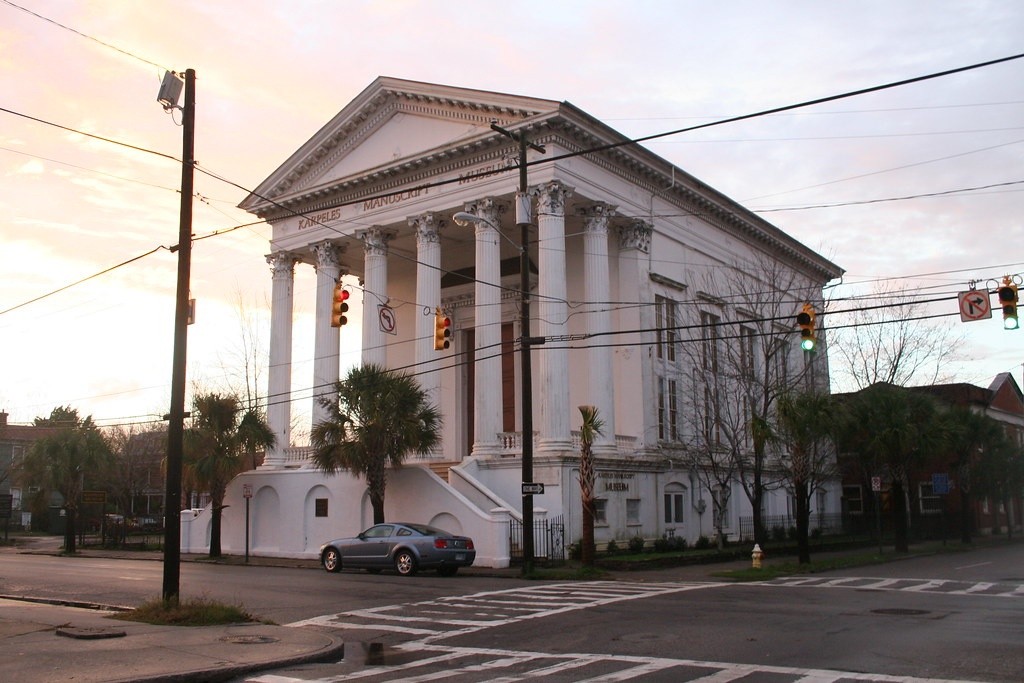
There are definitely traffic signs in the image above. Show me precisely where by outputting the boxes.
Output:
[522,481,544,495]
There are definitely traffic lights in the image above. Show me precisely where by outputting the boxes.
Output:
[330,287,349,328]
[797,305,816,351]
[434,316,450,351]
[998,285,1019,330]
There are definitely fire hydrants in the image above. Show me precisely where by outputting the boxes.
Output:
[751,543,764,568]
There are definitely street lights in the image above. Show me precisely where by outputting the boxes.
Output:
[453,211,534,573]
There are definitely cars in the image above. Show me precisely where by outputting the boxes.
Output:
[90,513,138,532]
[319,523,476,576]
